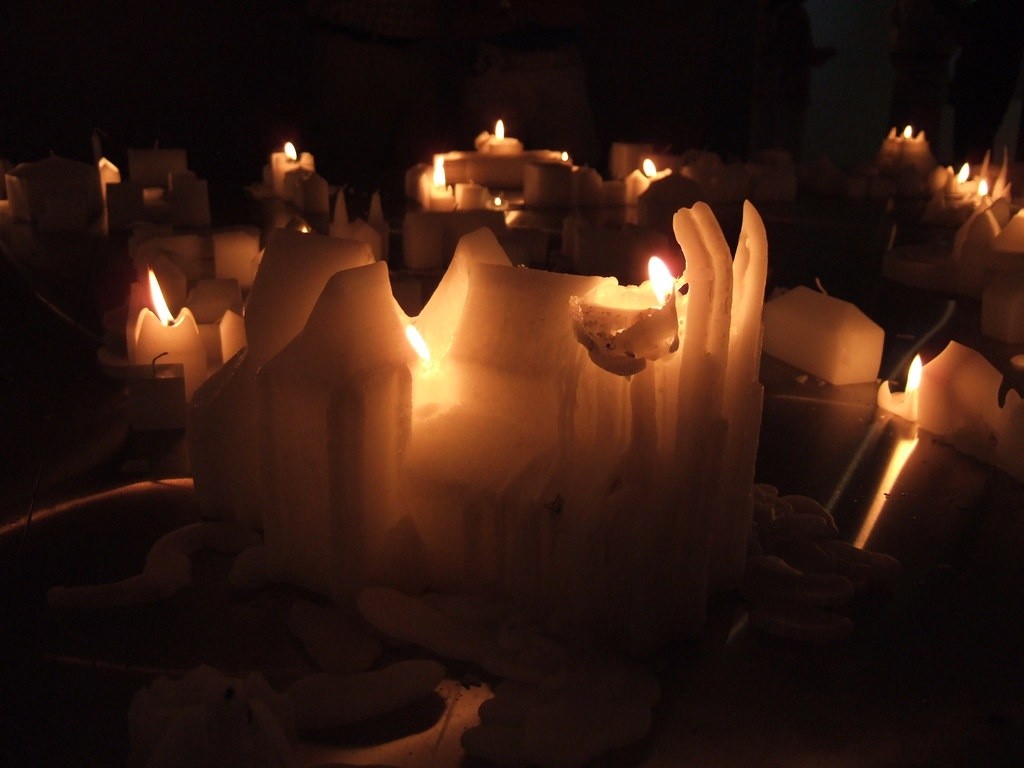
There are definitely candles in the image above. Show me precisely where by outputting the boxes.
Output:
[0,116,1024,661]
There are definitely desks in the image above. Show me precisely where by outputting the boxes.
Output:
[0,472,1024,768]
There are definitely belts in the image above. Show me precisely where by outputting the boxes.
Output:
[316,22,426,47]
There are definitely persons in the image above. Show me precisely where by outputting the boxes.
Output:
[302,0,603,191]
[883,0,1024,166]
[747,0,837,163]
